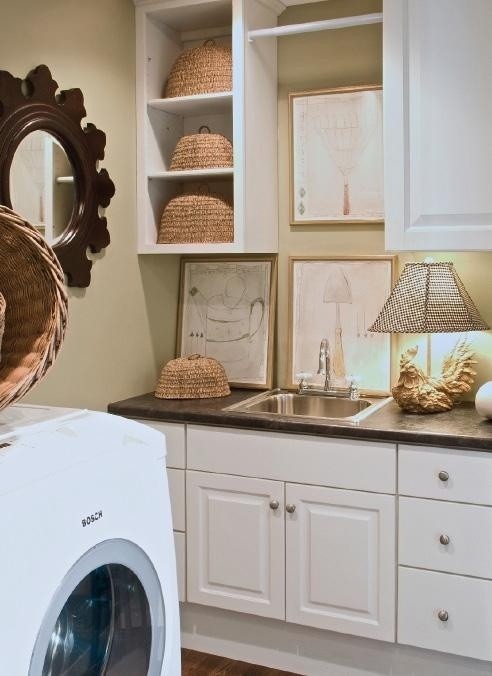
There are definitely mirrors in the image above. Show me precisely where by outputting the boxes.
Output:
[1,65,116,288]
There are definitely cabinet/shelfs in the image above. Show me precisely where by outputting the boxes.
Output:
[384,3,492,254]
[186,422,397,675]
[135,2,279,255]
[396,443,491,663]
[108,395,186,646]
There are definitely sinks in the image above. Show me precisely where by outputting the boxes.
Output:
[222,389,393,422]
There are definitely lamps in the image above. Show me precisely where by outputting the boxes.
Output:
[368,263,491,378]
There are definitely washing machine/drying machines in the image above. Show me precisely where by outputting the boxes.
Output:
[1,401,184,676]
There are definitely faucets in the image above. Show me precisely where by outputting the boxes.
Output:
[297,337,361,399]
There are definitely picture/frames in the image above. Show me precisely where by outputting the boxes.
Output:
[176,257,276,389]
[286,255,400,398]
[288,84,383,225]
